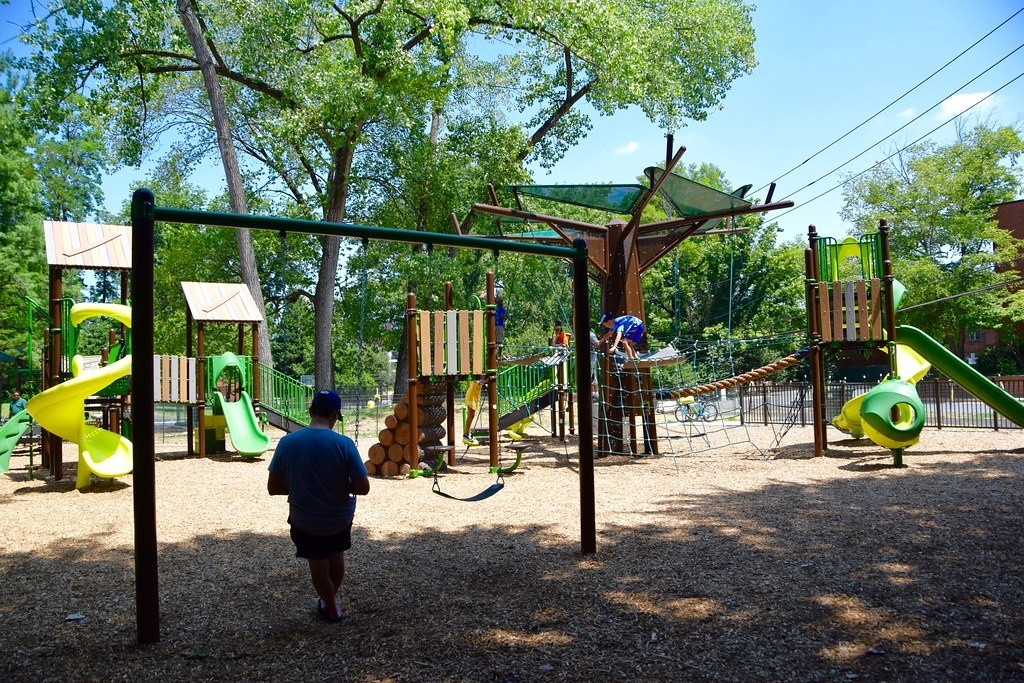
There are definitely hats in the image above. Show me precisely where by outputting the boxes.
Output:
[313,390,342,421]
[598,314,613,325]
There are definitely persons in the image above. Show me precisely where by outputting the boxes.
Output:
[267,390,370,620]
[462,381,482,445]
[598,315,644,359]
[551,326,568,389]
[495,297,509,356]
[589,325,599,402]
[9,391,27,419]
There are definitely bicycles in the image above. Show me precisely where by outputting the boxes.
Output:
[674,395,719,423]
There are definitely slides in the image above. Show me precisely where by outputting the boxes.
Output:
[212,390,271,455]
[833,328,931,449]
[897,323,1024,428]
[26,302,132,479]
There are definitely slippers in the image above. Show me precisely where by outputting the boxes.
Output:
[315,597,343,622]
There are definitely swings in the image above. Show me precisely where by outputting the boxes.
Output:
[280,244,367,553]
[428,254,505,503]
